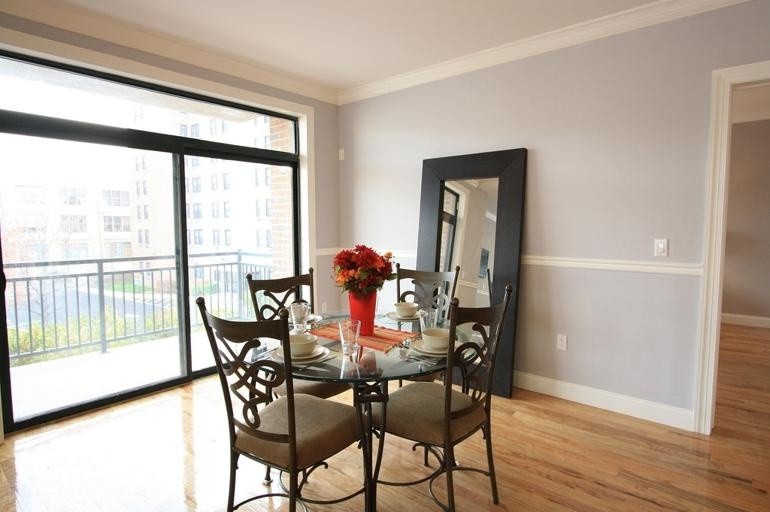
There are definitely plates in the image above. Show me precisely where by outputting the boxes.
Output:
[387,309,429,323]
[272,314,322,326]
[410,340,467,359]
[270,345,330,365]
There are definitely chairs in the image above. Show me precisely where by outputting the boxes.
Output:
[194,296,366,512]
[364,285,512,510]
[247,268,355,404]
[395,263,460,388]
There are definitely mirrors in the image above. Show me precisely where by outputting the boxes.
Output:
[410,148,527,399]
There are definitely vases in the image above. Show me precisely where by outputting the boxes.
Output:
[348,290,378,337]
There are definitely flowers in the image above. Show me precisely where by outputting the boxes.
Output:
[330,245,396,292]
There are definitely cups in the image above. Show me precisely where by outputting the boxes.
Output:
[289,301,312,329]
[336,318,362,355]
[340,352,360,380]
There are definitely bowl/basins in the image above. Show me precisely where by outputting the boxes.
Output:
[393,302,418,317]
[284,333,318,355]
[420,328,455,347]
